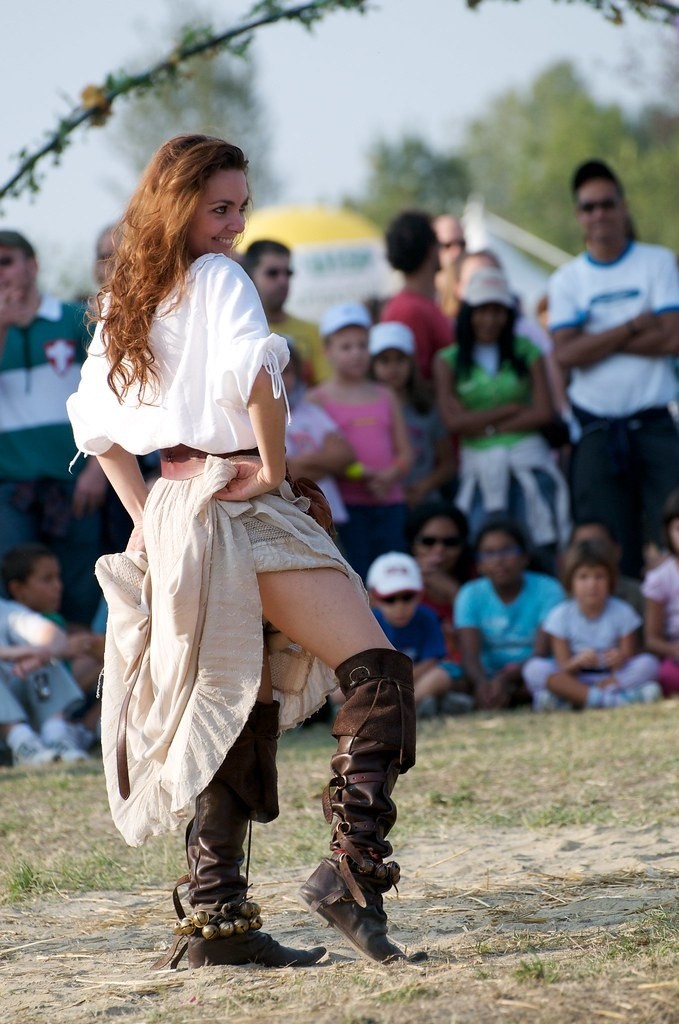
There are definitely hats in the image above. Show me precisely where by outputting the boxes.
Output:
[364,551,422,598]
[315,299,371,339]
[365,321,416,356]
[453,268,516,307]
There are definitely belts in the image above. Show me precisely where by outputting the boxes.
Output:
[629,406,669,424]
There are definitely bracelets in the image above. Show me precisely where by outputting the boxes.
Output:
[625,320,639,335]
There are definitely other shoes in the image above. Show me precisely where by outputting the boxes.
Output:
[13,740,59,767]
[47,735,87,763]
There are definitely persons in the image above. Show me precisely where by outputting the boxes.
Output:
[63,134,431,970]
[0,158,679,769]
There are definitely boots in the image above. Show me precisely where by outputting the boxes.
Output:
[297,647,430,969]
[149,700,327,975]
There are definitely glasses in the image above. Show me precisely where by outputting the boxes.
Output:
[576,197,616,214]
[382,590,418,604]
[416,531,462,548]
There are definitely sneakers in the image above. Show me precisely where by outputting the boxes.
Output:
[535,691,574,712]
[622,685,659,704]
